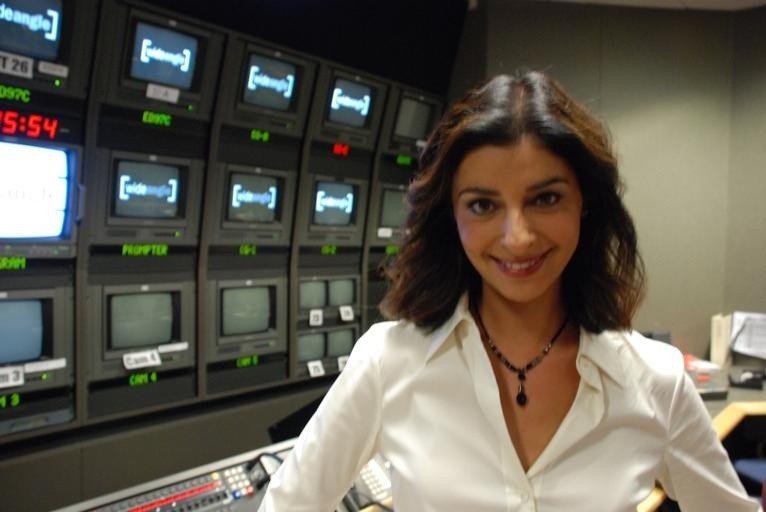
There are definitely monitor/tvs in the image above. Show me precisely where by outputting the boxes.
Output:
[128,20,200,91]
[326,76,373,128]
[100,282,191,361]
[0,141,68,239]
[0,0,65,61]
[114,160,182,218]
[0,285,68,372]
[394,97,432,139]
[242,52,298,111]
[296,271,360,366]
[217,277,280,344]
[227,173,279,223]
[381,189,408,228]
[313,181,355,226]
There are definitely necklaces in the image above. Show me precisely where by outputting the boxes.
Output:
[478,310,566,406]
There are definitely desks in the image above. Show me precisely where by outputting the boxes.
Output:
[640,362,766,512]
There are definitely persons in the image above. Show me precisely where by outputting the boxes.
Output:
[257,69,761,512]
